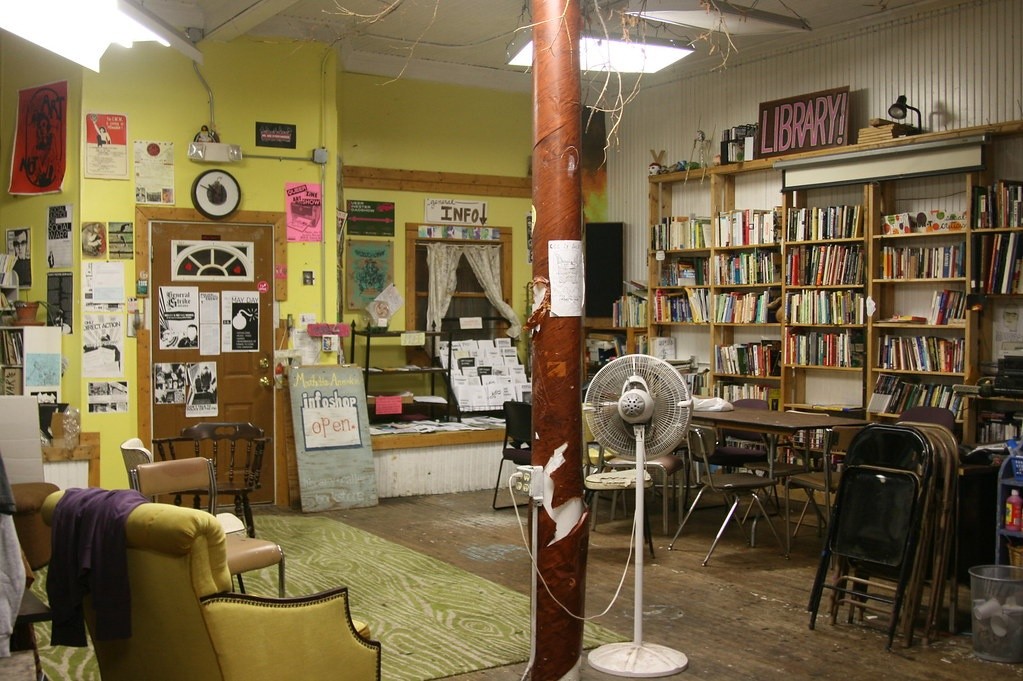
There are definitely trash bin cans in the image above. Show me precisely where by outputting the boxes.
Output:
[968,565,1023,662]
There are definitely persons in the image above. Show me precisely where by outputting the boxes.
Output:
[12,229,31,285]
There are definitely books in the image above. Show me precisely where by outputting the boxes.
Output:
[583,333,781,459]
[928,289,967,325]
[971,231,1023,295]
[879,241,966,279]
[876,335,965,373]
[972,178,1023,229]
[719,122,759,165]
[785,244,864,285]
[866,373,964,420]
[439,338,531,411]
[714,247,781,285]
[714,205,782,247]
[786,403,862,471]
[885,316,926,323]
[786,289,866,325]
[784,326,864,368]
[975,410,1023,445]
[612,213,712,327]
[786,204,863,242]
[713,287,781,324]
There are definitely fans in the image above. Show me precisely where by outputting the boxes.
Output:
[586,356,693,677]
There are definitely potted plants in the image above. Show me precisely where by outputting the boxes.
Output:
[7,298,60,330]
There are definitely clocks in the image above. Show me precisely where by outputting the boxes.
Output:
[190,169,241,219]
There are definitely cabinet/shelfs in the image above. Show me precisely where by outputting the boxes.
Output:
[994,444,1023,587]
[647,120,1023,525]
[0,285,61,403]
[349,321,453,424]
[585,326,647,381]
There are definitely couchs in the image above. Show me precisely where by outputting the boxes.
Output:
[41,490,380,681]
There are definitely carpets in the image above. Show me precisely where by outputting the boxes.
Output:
[29,516,632,681]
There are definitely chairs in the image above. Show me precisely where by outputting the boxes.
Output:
[492,399,960,650]
[130,457,285,598]
[120,437,158,504]
[151,422,272,538]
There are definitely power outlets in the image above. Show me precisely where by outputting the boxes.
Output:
[514,465,543,499]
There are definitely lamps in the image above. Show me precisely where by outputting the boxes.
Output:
[888,95,927,136]
[188,142,242,162]
[506,0,811,74]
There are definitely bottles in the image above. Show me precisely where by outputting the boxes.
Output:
[1005,489,1021,531]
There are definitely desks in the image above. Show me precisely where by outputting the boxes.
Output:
[590,395,873,555]
